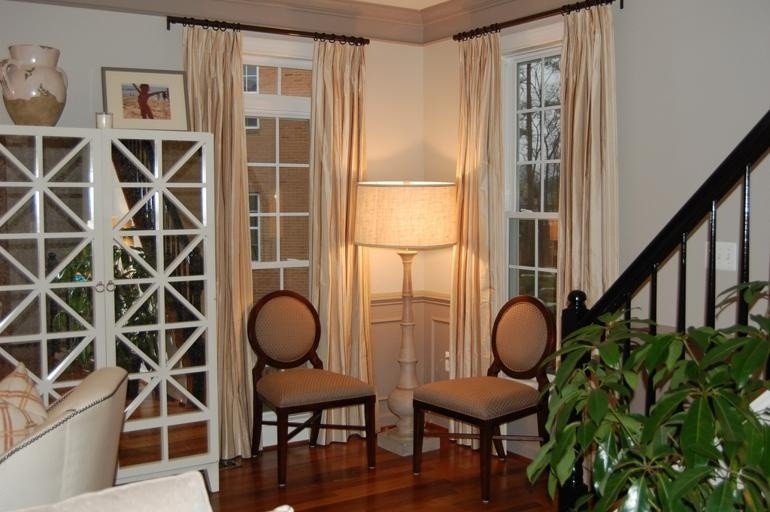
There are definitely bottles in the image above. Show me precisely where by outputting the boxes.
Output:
[1,44,67,127]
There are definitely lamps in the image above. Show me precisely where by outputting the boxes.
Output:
[348,177,461,463]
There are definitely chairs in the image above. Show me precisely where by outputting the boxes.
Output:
[407,291,556,506]
[244,291,381,490]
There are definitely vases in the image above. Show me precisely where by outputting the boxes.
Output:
[2,40,75,127]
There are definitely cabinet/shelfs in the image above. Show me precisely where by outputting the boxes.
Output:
[0,125,225,498]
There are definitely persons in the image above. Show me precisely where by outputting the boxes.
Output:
[130,83,164,119]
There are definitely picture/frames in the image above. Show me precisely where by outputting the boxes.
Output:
[101,61,193,133]
